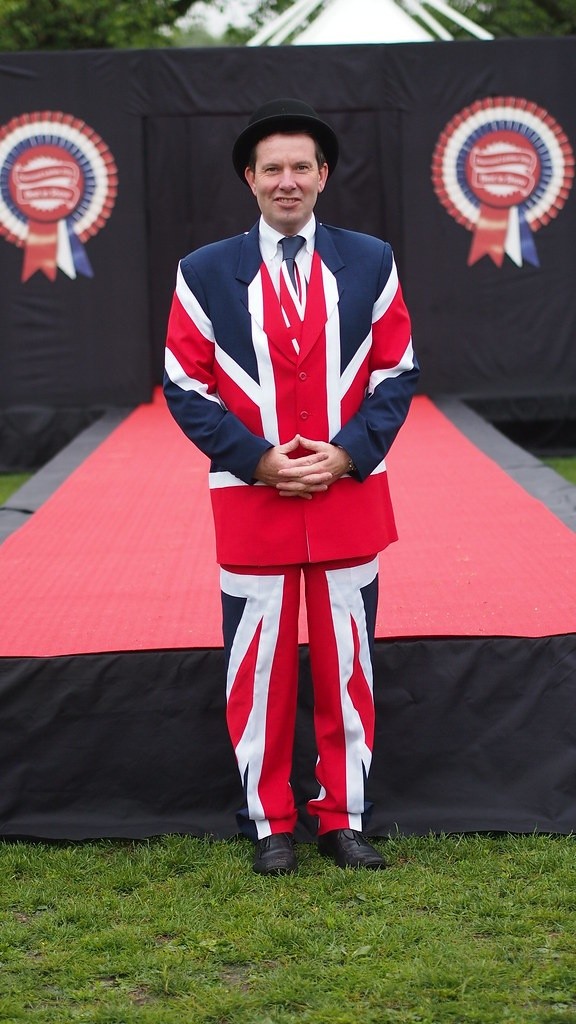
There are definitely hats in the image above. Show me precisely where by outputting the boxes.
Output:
[233,98,339,187]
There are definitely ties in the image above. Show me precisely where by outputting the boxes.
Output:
[279,237,309,354]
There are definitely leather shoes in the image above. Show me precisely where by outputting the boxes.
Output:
[319,827,387,870]
[254,832,297,874]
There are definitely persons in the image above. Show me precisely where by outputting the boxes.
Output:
[160,97,424,877]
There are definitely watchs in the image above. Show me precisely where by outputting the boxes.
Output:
[348,459,359,473]
